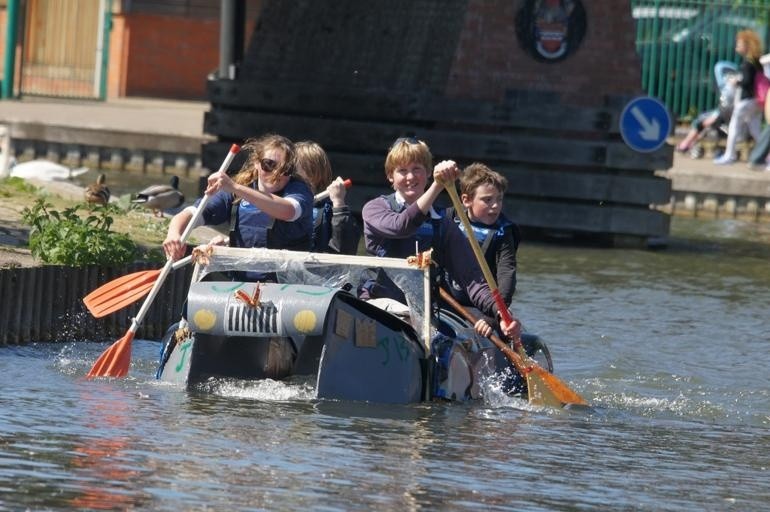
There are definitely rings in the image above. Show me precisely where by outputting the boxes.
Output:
[218,178,224,184]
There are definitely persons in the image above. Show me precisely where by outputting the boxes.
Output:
[294,141,360,256]
[677,26,770,174]
[442,161,519,308]
[362,136,524,342]
[161,134,314,261]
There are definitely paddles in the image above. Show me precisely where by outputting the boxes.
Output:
[436,171,561,410]
[83,179,352,317]
[88,144,240,376]
[432,285,588,405]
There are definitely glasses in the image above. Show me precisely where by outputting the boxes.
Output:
[258,158,293,178]
[394,137,418,145]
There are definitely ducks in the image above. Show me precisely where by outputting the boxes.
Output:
[131,176,185,218]
[84,173,109,215]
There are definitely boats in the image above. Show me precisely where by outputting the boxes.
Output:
[155,244,554,402]
